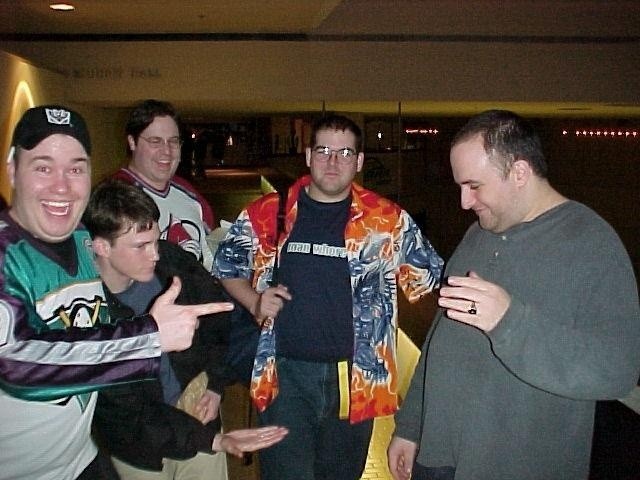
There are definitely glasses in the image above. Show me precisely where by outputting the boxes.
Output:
[310,144,360,161]
[137,133,185,149]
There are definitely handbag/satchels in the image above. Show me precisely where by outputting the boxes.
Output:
[174,370,210,420]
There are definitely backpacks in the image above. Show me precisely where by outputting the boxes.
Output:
[207,179,291,389]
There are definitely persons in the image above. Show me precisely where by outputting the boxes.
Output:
[81,177,292,479]
[112,100,221,283]
[386,106,639,480]
[211,114,446,479]
[0,103,234,479]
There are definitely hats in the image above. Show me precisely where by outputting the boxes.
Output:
[11,104,92,154]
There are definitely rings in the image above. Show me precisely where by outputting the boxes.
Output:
[467,301,478,316]
